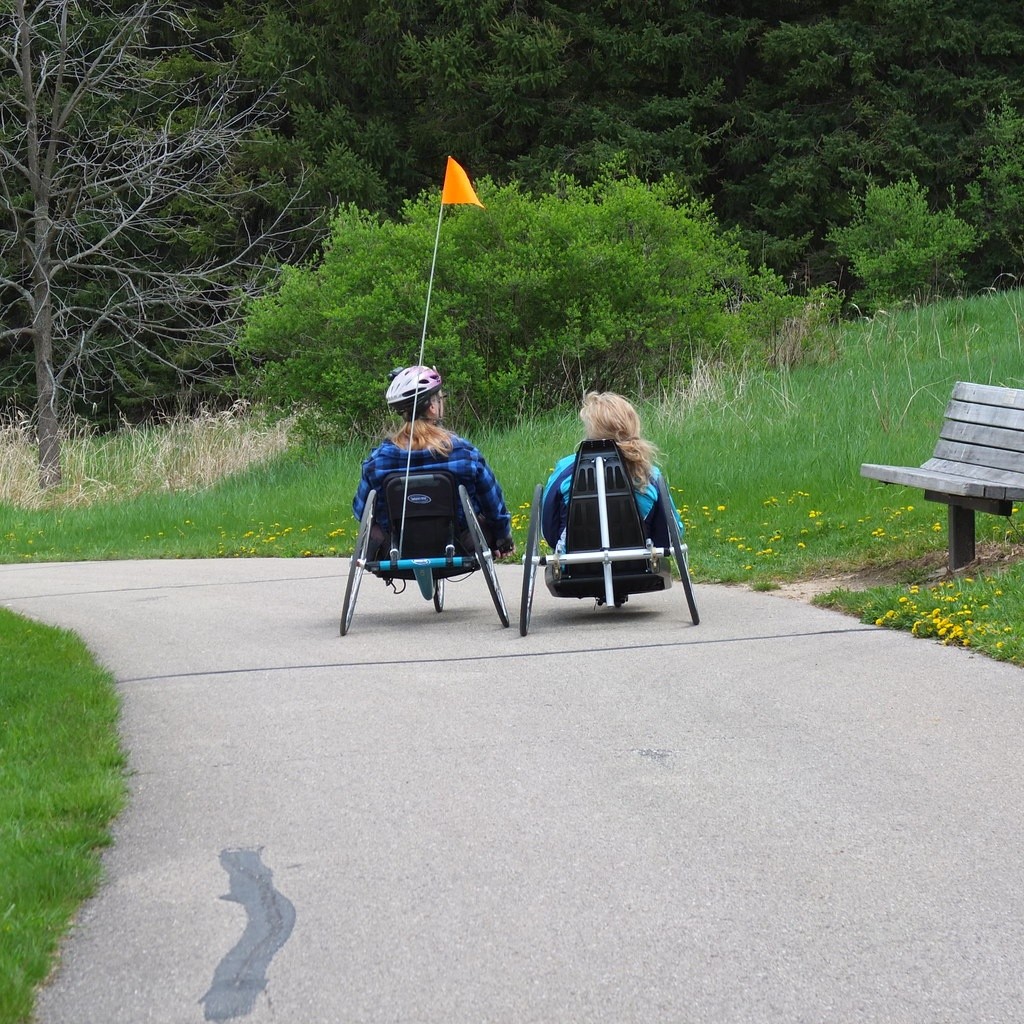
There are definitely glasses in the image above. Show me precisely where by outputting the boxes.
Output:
[431,391,448,404]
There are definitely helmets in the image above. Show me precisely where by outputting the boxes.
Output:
[386,366,442,415]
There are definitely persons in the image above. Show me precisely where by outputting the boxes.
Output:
[351,367,513,561]
[540,391,683,579]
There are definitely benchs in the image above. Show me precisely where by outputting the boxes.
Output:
[860,381,1023,570]
